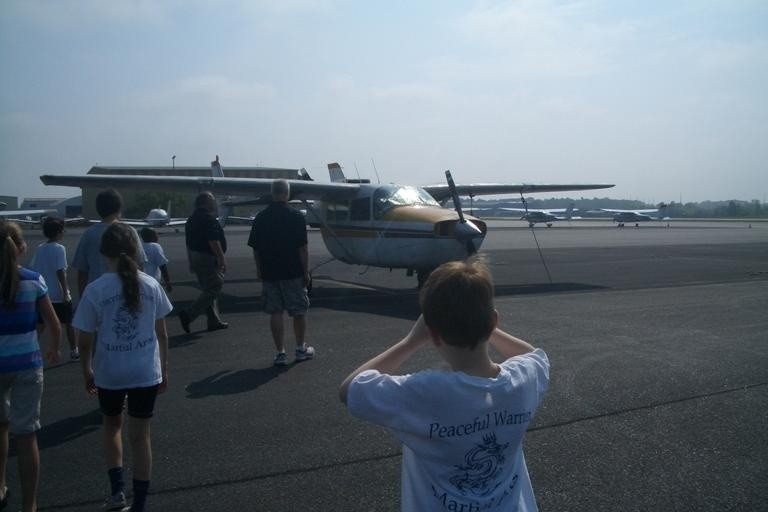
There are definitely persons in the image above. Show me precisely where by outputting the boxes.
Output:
[67,186,150,303]
[176,187,231,334]
[247,178,318,369]
[70,220,176,511]
[0,217,63,510]
[334,249,554,510]
[138,225,173,290]
[25,213,82,366]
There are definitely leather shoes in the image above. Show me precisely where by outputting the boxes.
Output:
[208,322,229,330]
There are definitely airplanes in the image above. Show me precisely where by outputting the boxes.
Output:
[84,207,190,229]
[496,206,583,228]
[0,208,57,218]
[39,163,615,301]
[592,204,670,228]
[5,214,83,226]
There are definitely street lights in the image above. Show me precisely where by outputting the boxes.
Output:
[171,154,176,174]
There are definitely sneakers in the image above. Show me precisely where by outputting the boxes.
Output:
[101,492,126,511]
[296,343,314,360]
[274,353,287,365]
